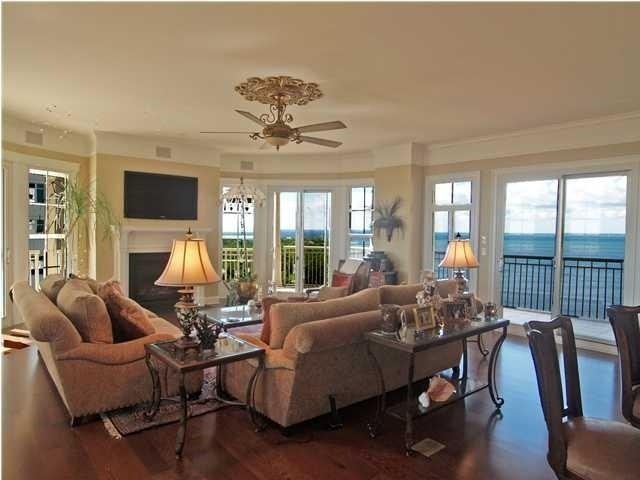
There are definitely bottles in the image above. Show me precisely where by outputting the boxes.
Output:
[439,316,444,325]
[31,254,35,275]
[38,256,44,273]
[35,183,44,201]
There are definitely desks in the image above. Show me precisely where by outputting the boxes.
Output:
[363,314,510,456]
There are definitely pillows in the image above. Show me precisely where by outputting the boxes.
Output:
[40,274,156,344]
[260,271,458,348]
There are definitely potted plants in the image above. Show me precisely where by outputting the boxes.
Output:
[193,314,223,355]
[234,272,257,297]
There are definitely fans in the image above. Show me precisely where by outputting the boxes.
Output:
[200,75,347,148]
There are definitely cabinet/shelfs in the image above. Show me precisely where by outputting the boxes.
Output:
[28,169,70,293]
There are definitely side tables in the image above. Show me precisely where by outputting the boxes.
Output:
[143,332,269,460]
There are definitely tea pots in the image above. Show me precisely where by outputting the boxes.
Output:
[375,303,402,335]
[480,302,503,321]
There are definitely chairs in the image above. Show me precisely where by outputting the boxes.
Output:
[303,259,369,303]
[523,316,640,480]
[606,305,640,428]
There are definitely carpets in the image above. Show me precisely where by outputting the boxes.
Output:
[1,329,32,358]
[99,366,235,440]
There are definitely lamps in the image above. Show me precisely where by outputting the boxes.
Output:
[154,227,222,357]
[437,232,480,294]
[216,176,267,275]
[262,126,292,150]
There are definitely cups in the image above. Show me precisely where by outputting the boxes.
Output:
[248,307,254,313]
[37,216,44,234]
[248,300,254,306]
[255,302,262,308]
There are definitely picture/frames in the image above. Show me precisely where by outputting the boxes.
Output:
[442,301,467,319]
[412,304,436,331]
[448,294,477,316]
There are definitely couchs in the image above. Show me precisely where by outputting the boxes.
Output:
[219,278,483,435]
[9,279,203,427]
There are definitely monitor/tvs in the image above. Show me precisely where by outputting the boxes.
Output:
[124,171,198,219]
